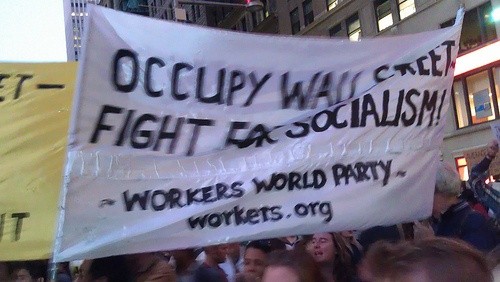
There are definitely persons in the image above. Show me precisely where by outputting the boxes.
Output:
[1,136,500,282]
